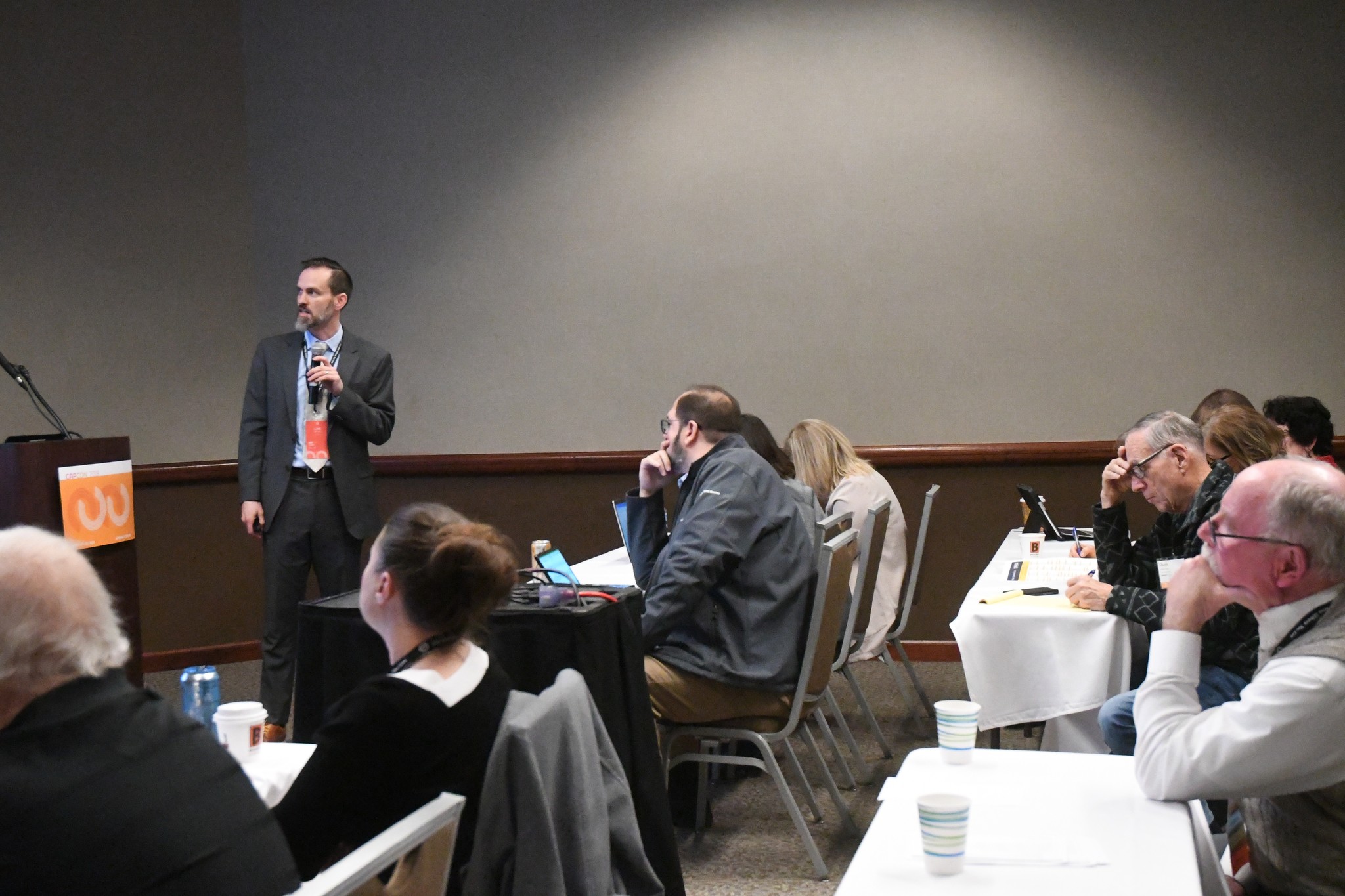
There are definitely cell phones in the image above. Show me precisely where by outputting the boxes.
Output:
[1003,587,1059,596]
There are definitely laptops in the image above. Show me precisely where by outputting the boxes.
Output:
[613,497,632,562]
[1016,482,1095,541]
[534,545,635,589]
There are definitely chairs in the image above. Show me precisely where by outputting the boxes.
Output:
[887,481,943,743]
[831,497,903,784]
[655,527,861,882]
[811,509,869,824]
[284,790,467,896]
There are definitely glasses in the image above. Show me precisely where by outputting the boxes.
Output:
[1208,517,1310,570]
[1208,454,1232,470]
[1126,444,1175,479]
[661,420,702,434]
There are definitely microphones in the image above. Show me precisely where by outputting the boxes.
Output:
[0,351,29,390]
[309,342,326,404]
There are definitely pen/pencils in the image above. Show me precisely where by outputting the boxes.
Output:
[1087,570,1095,577]
[1072,527,1082,557]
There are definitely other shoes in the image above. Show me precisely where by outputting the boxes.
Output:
[708,741,762,780]
[668,790,713,828]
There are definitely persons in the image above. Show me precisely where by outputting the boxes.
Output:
[1132,457,1345,896]
[1191,387,1257,469]
[1198,405,1284,476]
[786,419,908,663]
[268,501,518,896]
[238,256,396,742]
[729,413,855,779]
[1065,411,1258,840]
[1264,395,1339,468]
[0,525,302,896]
[624,383,816,838]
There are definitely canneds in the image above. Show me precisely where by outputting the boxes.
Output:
[531,539,551,582]
[180,664,221,741]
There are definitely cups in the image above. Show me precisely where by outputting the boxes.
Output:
[934,700,982,764]
[916,793,971,876]
[1020,495,1046,532]
[212,701,268,765]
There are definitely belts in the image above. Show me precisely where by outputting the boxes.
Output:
[290,466,333,479]
[263,720,286,743]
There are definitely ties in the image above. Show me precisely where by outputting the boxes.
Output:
[301,342,329,472]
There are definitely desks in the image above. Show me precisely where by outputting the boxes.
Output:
[525,545,645,600]
[948,521,1149,748]
[832,745,1226,896]
[292,583,688,896]
